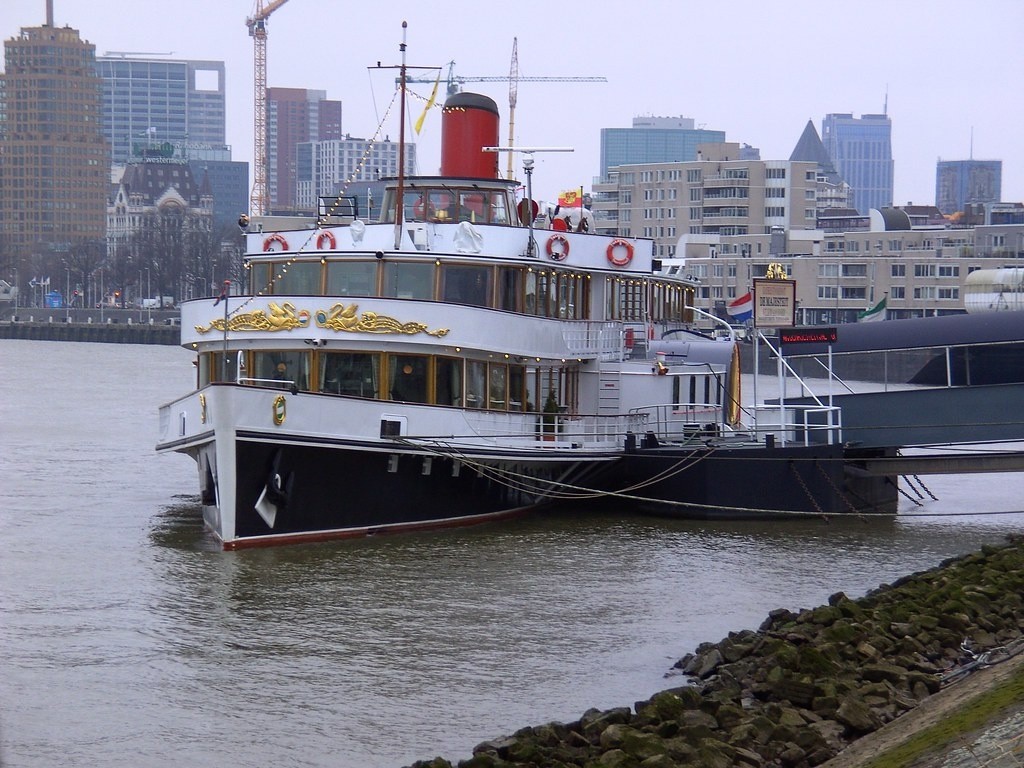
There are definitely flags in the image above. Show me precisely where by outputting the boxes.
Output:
[28,277,50,287]
[855,299,886,324]
[726,293,753,321]
[415,75,442,135]
[559,188,581,207]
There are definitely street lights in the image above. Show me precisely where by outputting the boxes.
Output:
[144,267,151,323]
[197,258,218,296]
[12,268,17,316]
[99,267,104,323]
[138,270,143,323]
[64,268,69,318]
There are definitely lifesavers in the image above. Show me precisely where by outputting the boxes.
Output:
[607,239,633,266]
[546,234,569,260]
[263,234,288,251]
[414,198,436,220]
[317,231,335,249]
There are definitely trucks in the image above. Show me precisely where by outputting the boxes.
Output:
[162,317,181,328]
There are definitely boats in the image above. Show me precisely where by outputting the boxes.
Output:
[155,21,843,552]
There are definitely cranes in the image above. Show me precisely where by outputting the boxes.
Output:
[244,1,294,212]
[396,34,611,173]
